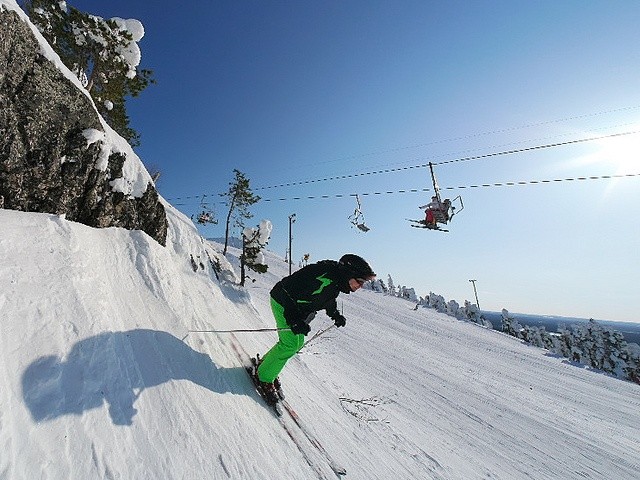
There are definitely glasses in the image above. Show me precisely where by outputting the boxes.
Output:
[354,278,364,285]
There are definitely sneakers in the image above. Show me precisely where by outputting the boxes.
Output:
[254,370,279,396]
[274,377,281,389]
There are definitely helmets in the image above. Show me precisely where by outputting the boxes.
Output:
[338,254,376,294]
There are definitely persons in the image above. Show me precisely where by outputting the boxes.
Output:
[199,211,209,220]
[416,197,451,224]
[254,254,376,402]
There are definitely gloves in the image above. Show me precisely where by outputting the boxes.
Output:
[290,320,311,336]
[330,311,346,327]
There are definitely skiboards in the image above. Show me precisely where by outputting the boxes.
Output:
[406,218,449,233]
[229,330,347,480]
